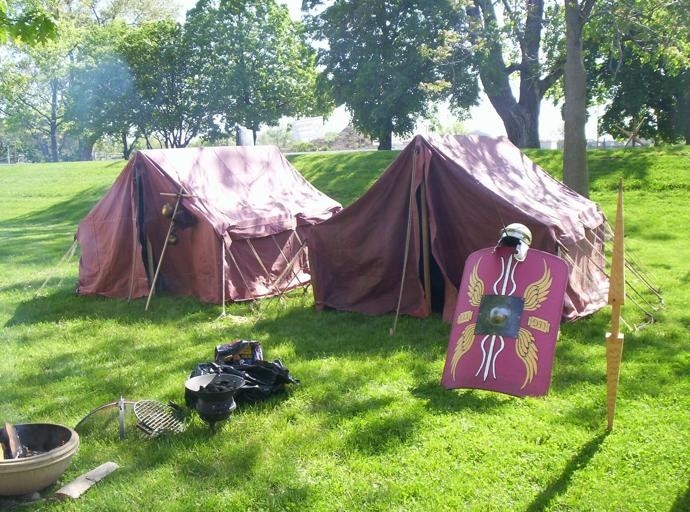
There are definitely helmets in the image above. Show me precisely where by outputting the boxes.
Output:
[492,222,533,262]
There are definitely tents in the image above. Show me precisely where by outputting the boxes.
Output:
[32,144,343,316]
[262,134,664,326]
